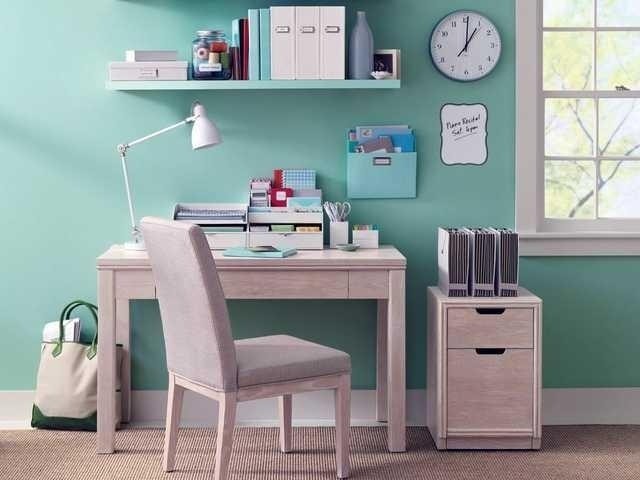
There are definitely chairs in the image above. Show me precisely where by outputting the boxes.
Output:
[137,217,351,480]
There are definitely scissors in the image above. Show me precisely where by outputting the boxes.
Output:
[335,202,352,222]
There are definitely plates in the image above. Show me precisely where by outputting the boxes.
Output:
[335,244,360,251]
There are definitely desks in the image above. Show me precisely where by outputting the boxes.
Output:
[95,243,406,453]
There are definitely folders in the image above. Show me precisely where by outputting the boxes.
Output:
[270,6,345,80]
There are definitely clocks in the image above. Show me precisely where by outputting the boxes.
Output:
[428,9,502,83]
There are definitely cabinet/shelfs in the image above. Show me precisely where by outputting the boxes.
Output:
[426,285,544,451]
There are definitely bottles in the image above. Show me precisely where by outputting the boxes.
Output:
[348,11,375,79]
[191,30,232,79]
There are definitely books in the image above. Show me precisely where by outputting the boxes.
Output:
[126,49,177,62]
[223,246,297,258]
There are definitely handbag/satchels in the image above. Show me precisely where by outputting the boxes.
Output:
[31,300,129,432]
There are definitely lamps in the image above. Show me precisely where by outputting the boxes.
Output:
[118,97,222,248]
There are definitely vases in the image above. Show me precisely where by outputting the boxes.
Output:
[348,11,375,80]
